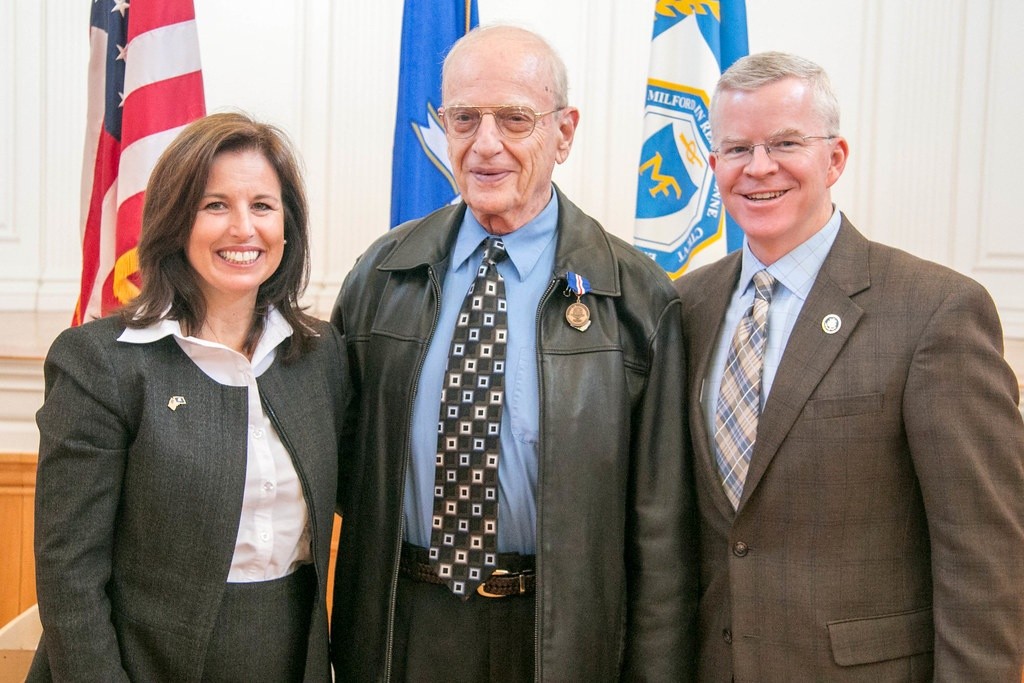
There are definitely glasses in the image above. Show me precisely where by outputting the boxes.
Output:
[714,131,834,160]
[439,104,567,140]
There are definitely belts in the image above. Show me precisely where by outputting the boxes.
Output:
[400,548,538,598]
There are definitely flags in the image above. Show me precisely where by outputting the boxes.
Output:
[68,2,208,327]
[631,0,748,278]
[389,1,502,230]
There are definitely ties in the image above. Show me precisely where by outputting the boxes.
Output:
[712,268,780,514]
[427,237,509,603]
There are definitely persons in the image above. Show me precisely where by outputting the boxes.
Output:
[671,50,1018,683]
[328,23,689,682]
[22,110,352,683]
